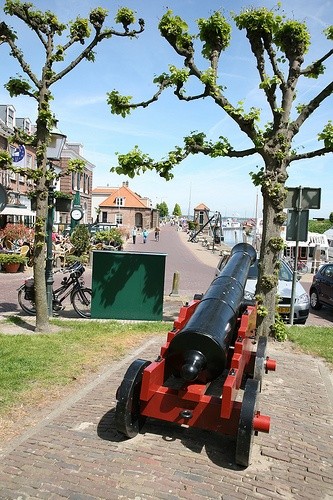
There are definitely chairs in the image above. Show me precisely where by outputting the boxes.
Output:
[16,245,30,269]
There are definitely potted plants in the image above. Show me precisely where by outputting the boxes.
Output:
[26,191,37,211]
[0,253,25,273]
[54,190,73,213]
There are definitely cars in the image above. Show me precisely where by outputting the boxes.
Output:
[308,263,333,323]
[213,251,311,325]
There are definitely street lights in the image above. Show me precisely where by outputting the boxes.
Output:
[44,114,53,319]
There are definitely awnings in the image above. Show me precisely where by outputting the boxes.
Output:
[0,207,37,217]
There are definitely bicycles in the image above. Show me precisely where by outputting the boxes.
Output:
[15,260,93,318]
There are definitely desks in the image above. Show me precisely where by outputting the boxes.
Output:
[52,250,64,267]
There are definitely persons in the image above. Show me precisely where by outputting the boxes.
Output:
[170,218,173,226]
[142,229,149,243]
[155,227,160,241]
[52,231,57,241]
[22,238,31,259]
[131,226,136,244]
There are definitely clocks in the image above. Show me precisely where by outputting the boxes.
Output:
[72,210,81,219]
